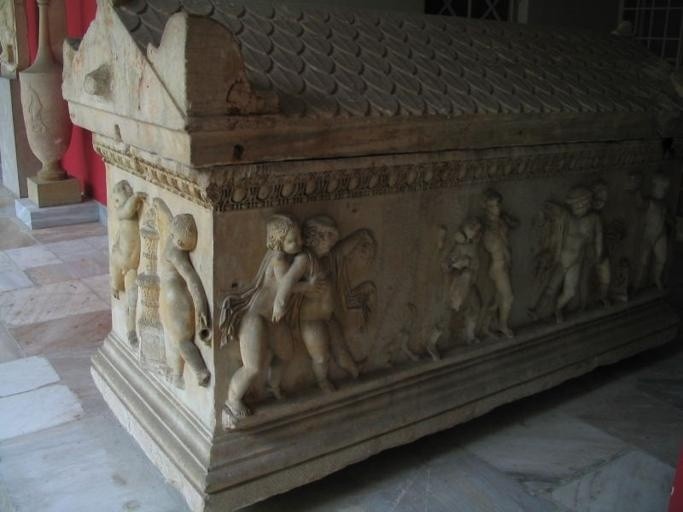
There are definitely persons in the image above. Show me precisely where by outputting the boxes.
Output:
[151,195,213,392]
[425,186,522,358]
[108,178,147,349]
[223,210,373,425]
[537,167,675,326]
